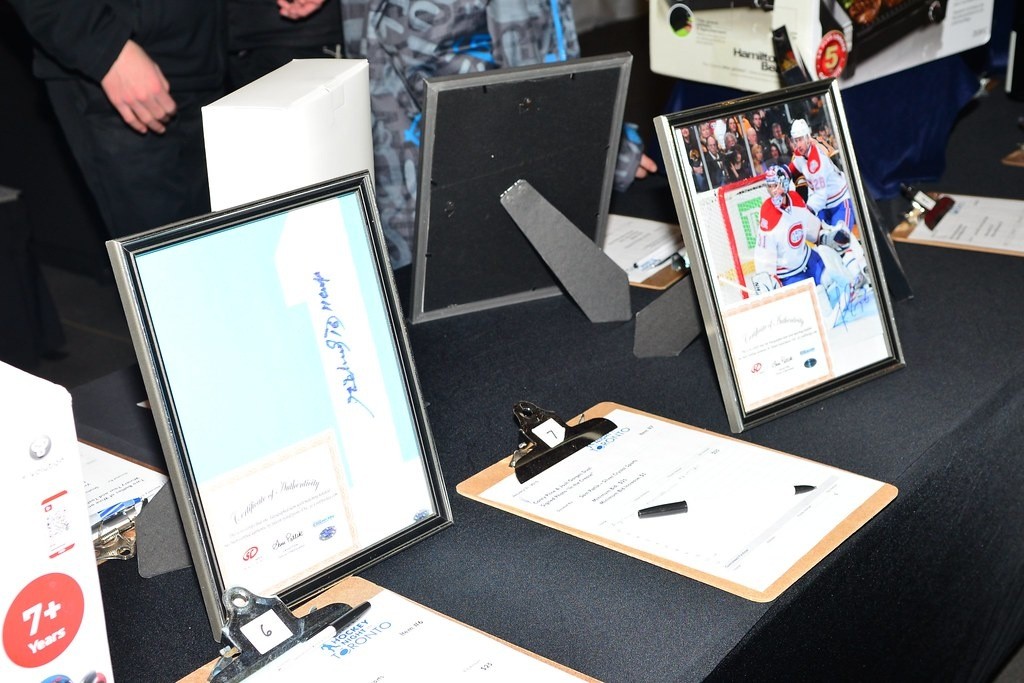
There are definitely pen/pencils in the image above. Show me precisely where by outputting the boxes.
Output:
[88,497,143,523]
[633,233,683,268]
[637,484,817,518]
[271,600,371,670]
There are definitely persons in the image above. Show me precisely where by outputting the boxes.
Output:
[680,94,873,310]
[338,1,660,269]
[7,1,348,238]
[0,128,66,373]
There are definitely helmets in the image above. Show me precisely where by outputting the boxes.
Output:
[790,119,812,145]
[765,165,789,194]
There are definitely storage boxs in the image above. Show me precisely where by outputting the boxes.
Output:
[0,359,114,683]
[650,0,995,94]
[201,56,376,212]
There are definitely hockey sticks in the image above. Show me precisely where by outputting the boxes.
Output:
[717,275,755,294]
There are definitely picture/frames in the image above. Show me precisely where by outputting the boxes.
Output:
[412,53,634,324]
[107,170,454,645]
[655,76,906,432]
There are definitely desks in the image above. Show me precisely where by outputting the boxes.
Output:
[65,86,1024,683]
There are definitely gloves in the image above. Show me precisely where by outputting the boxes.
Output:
[751,272,783,295]
[819,219,850,252]
